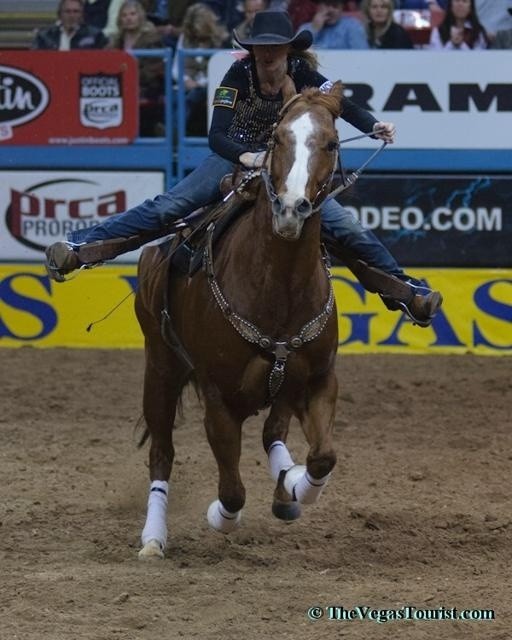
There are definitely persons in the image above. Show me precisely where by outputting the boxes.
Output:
[25,0,512,136]
[43,7,444,332]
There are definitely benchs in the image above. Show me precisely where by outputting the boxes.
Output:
[0,12,496,109]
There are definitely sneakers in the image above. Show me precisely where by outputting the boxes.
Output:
[43,243,71,280]
[401,290,443,328]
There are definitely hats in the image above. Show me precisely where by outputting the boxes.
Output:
[232,10,314,53]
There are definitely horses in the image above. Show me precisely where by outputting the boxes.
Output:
[134,74,344,565]
